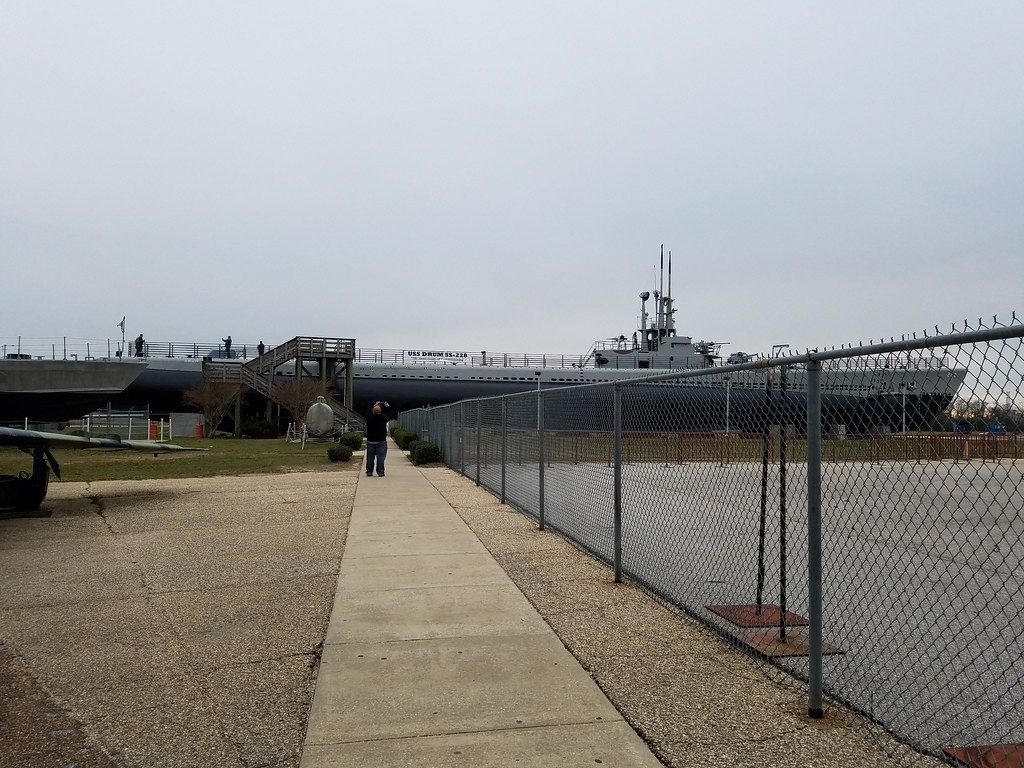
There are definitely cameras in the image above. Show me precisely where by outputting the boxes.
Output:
[380,402,385,406]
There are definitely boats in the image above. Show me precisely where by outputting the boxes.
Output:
[0,335,150,430]
[108,244,968,437]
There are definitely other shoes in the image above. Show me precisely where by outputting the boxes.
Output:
[367,473,373,477]
[378,474,385,477]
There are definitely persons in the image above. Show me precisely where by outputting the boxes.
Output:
[257,341,265,356]
[134,334,144,357]
[365,401,393,477]
[222,336,232,359]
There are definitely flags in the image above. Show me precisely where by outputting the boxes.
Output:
[116,319,125,333]
